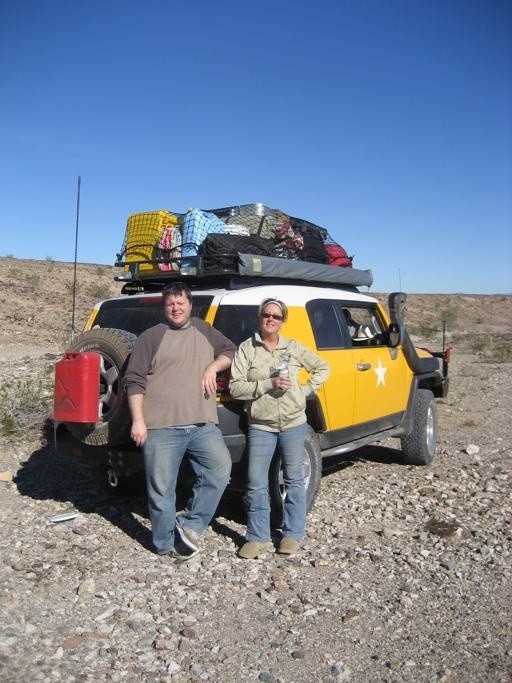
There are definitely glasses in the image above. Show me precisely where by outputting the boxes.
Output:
[260,312,284,320]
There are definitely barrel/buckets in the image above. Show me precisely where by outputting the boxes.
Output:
[181,209,222,267]
[54,352,101,423]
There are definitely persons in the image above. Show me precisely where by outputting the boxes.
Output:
[228,296,330,559]
[123,277,237,562]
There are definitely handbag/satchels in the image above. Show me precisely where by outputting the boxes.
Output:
[124,209,352,273]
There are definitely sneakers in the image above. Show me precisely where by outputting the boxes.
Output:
[278,537,298,554]
[238,540,267,559]
[165,518,198,560]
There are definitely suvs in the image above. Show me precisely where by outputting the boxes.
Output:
[53,174,451,524]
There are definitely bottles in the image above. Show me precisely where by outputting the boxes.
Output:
[276,362,290,392]
